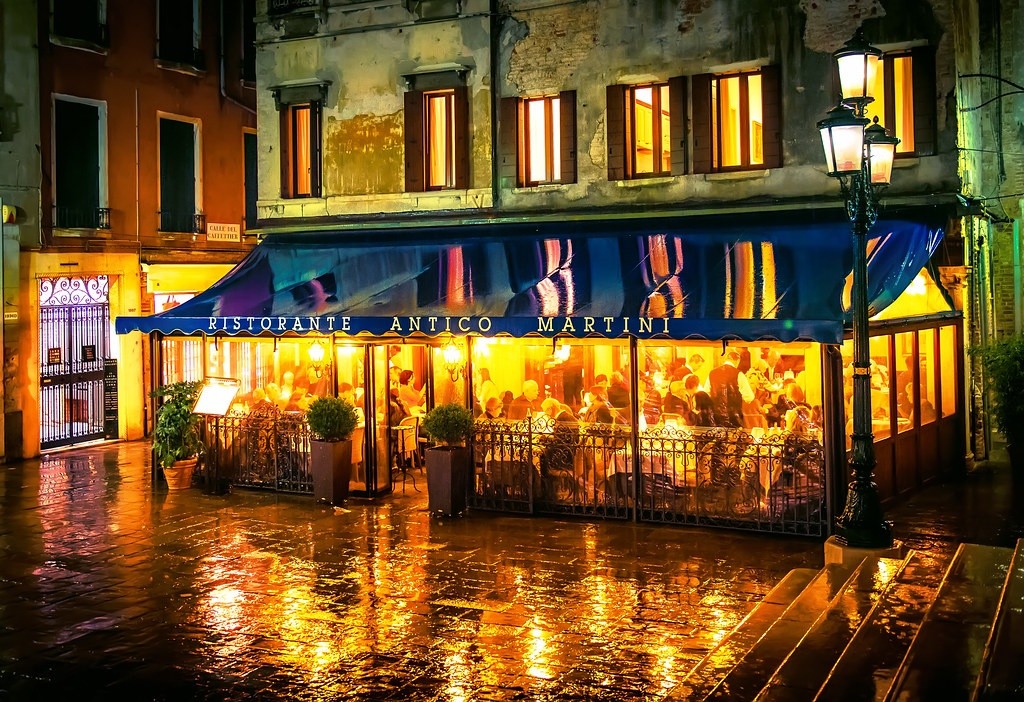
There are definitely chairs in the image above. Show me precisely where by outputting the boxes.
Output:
[389,417,420,469]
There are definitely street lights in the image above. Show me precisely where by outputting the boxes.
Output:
[814,25,900,549]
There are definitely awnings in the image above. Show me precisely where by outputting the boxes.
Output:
[114,209,945,342]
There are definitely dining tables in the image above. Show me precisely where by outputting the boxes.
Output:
[465,398,902,516]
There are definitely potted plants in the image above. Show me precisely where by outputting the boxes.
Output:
[421,405,483,516]
[966,334,1024,488]
[307,396,358,506]
[141,381,205,490]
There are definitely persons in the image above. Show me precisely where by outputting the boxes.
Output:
[227,345,936,502]
